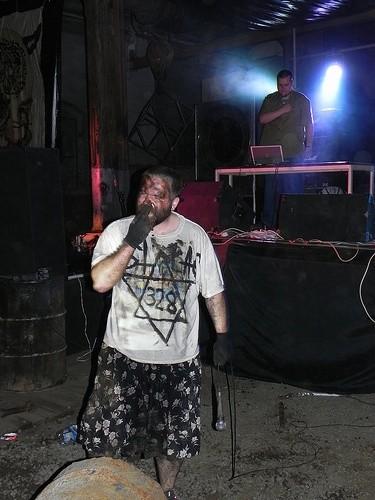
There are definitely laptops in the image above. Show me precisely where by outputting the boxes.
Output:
[250,145,290,165]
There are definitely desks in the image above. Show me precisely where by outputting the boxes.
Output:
[215,160,375,225]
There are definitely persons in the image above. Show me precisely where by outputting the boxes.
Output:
[76,164,231,500]
[257,70,312,229]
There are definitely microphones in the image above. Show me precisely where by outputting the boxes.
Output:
[215,384,226,431]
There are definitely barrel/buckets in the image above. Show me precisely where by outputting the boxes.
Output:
[0,254,68,393]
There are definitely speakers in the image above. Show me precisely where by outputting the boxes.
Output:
[277,193,375,242]
[177,182,253,231]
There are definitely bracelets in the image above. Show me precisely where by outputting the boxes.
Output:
[306,146,310,147]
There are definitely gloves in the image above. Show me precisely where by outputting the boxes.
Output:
[122,202,160,251]
[212,332,233,367]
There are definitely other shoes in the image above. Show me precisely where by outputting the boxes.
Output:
[164,489,181,500]
